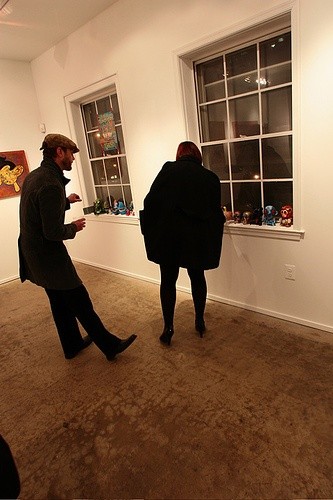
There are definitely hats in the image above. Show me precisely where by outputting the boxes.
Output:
[40,134,79,153]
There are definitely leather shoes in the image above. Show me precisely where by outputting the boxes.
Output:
[65,336,93,359]
[106,335,136,361]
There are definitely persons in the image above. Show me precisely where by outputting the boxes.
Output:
[18,134,137,362]
[141,141,227,348]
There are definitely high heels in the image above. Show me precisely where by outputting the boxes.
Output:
[195,319,205,338]
[159,325,173,345]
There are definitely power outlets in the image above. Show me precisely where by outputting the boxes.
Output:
[285,265,296,279]
